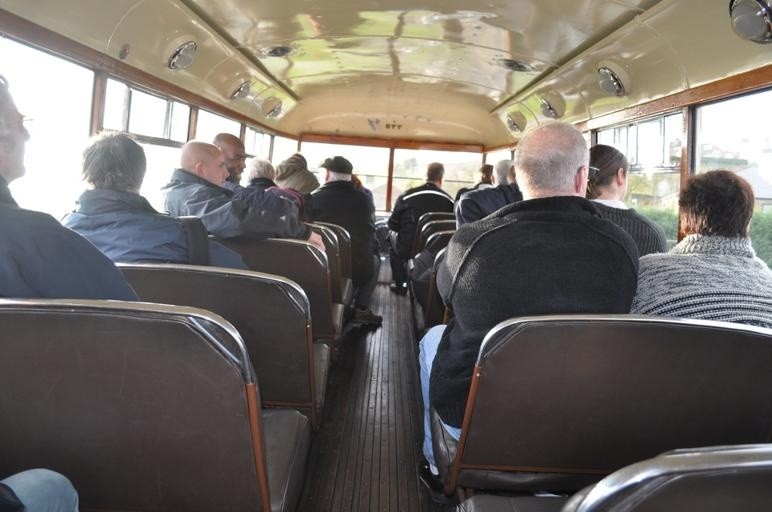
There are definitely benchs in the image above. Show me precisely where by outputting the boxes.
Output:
[296,221,343,295]
[326,221,353,281]
[112,258,324,414]
[413,212,457,326]
[0,298,310,512]
[239,235,340,340]
[448,437,772,510]
[429,310,767,496]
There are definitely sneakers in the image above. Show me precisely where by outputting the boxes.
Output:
[352,307,383,324]
[419,460,449,504]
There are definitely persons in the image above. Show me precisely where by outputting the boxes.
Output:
[387,160,521,296]
[417,119,639,504]
[46,128,248,272]
[586,143,667,258]
[625,168,772,332]
[0,75,140,303]
[159,139,326,249]
[249,154,383,324]
[0,467,79,511]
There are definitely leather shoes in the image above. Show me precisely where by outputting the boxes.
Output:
[391,283,408,296]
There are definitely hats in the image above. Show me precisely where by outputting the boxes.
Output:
[321,156,353,174]
